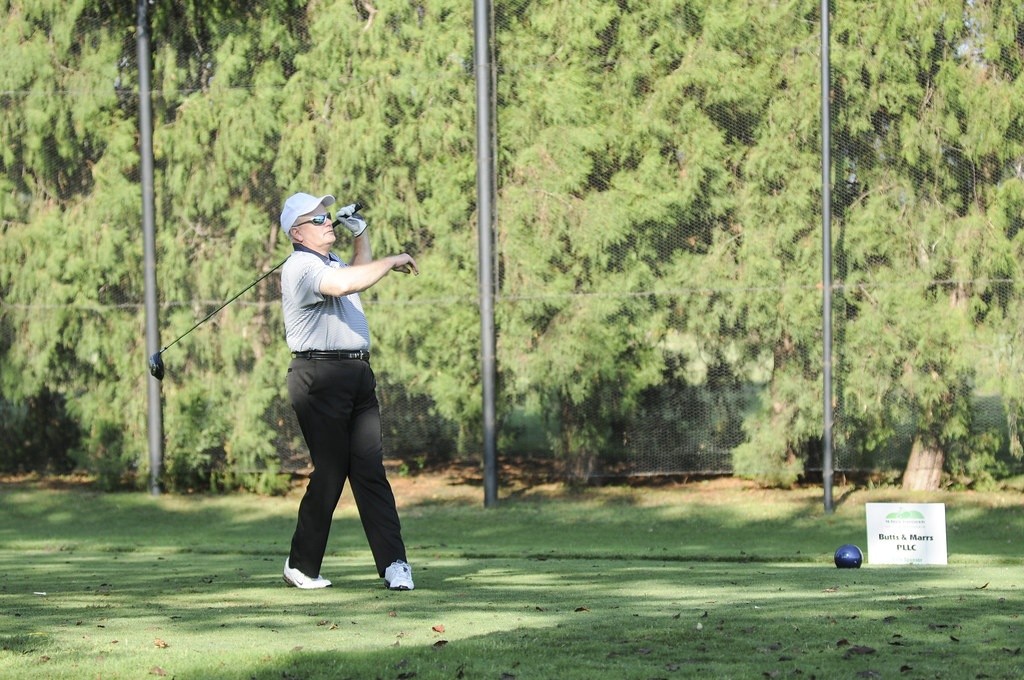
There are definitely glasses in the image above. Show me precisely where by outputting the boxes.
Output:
[292,212,332,227]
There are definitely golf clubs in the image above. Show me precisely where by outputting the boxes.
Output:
[148,201,364,381]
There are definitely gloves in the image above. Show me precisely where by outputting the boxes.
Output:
[336,204,368,237]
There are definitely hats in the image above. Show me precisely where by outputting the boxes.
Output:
[280,193,336,236]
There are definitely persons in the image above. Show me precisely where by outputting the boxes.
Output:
[280,191,418,590]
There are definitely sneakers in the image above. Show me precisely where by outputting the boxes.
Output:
[283,556,332,589]
[384,558,415,590]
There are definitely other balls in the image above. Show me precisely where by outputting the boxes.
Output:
[834,543,864,569]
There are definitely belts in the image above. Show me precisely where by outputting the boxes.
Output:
[292,349,369,358]
